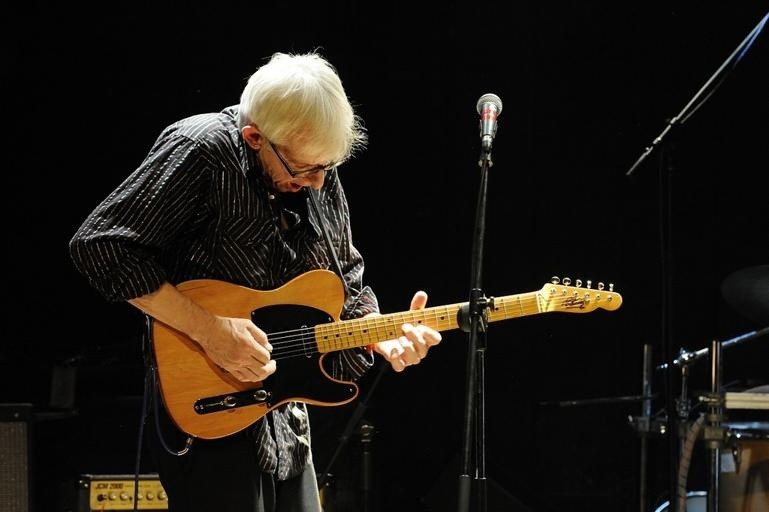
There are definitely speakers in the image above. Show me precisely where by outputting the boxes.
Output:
[0,402,39,511]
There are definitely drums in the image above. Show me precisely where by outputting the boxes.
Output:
[708,422,769,511]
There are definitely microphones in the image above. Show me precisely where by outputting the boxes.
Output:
[476,93,503,152]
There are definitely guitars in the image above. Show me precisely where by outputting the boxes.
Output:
[149,270,623,440]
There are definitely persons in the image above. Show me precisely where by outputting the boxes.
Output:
[68,53,442,512]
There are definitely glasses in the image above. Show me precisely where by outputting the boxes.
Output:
[249,122,344,180]
